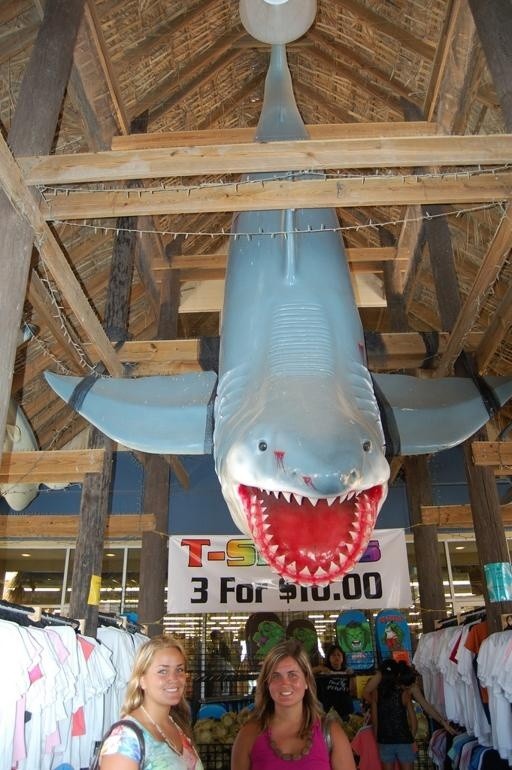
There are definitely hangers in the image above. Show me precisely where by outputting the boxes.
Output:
[0,601,80,633]
[430,607,487,631]
[97,613,144,634]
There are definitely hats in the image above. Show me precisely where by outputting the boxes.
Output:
[375,659,400,673]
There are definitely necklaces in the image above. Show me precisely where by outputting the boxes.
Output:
[140,705,202,770]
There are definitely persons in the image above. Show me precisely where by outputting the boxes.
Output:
[98,635,205,770]
[311,645,357,723]
[351,659,458,770]
[231,640,355,770]
[210,630,230,661]
